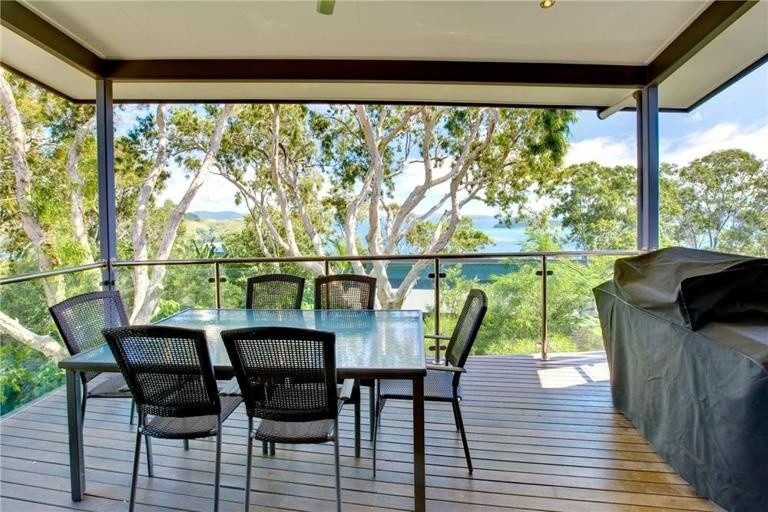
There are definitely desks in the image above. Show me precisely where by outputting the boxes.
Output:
[58,309,427,510]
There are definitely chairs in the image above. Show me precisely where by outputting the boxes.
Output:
[371,289,487,476]
[246,274,382,442]
[221,326,346,511]
[50,289,189,477]
[102,324,245,510]
